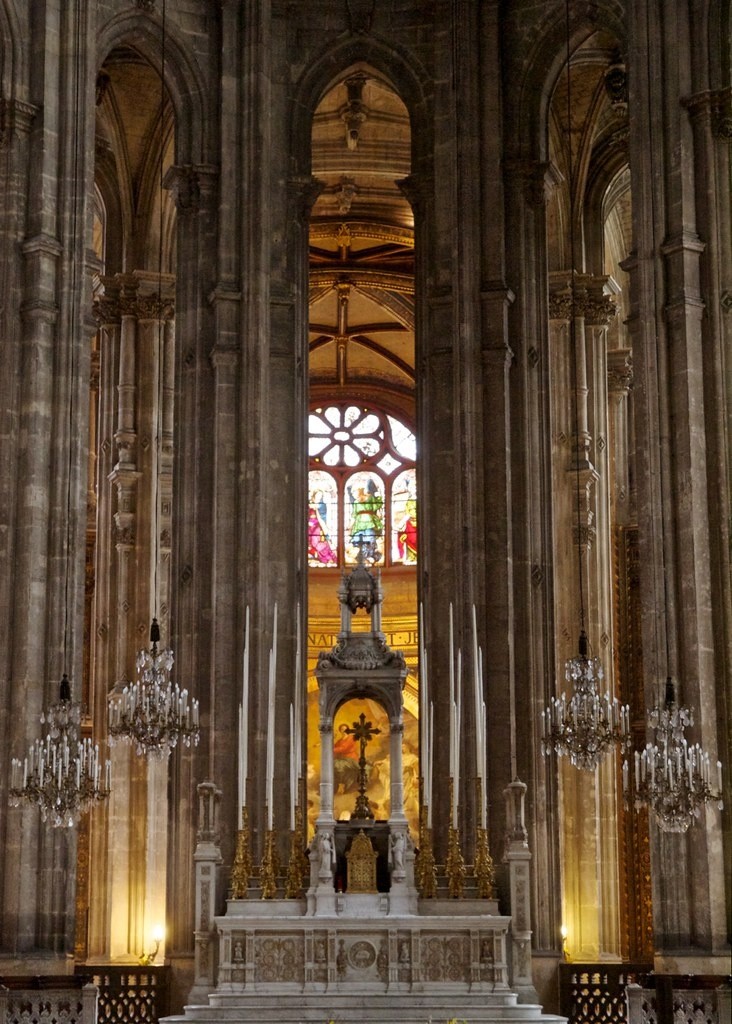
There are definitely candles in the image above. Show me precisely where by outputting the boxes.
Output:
[289,602,302,830]
[419,602,435,828]
[237,605,250,827]
[473,603,488,832]
[266,604,279,830]
[449,602,462,830]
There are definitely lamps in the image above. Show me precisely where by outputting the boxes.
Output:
[108,0,205,755]
[11,0,118,828]
[622,0,727,837]
[541,2,632,772]
